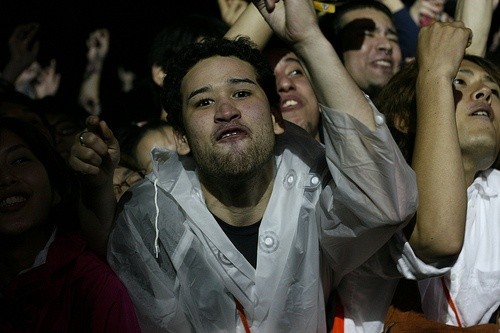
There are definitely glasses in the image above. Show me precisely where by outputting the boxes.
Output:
[112,171,144,196]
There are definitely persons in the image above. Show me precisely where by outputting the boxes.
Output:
[68,120,174,255]
[217,0,500,333]
[0,117,140,333]
[0,23,217,138]
[107,0,419,333]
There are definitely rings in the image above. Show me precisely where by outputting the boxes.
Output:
[79,132,86,142]
